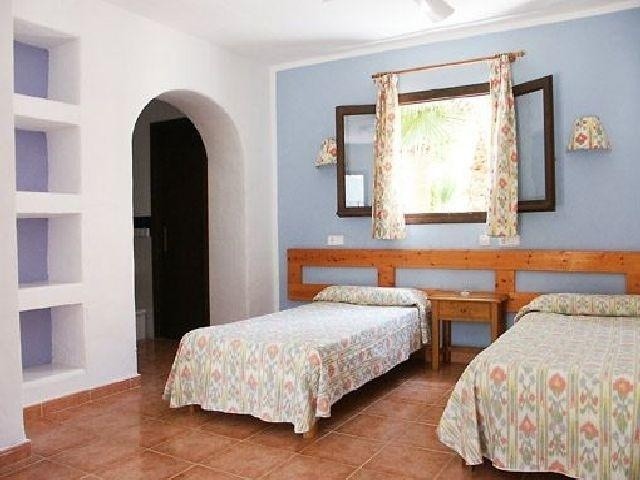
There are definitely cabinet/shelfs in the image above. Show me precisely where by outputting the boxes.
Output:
[14,18,91,411]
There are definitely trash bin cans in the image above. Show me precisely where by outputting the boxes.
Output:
[136,309,147,339]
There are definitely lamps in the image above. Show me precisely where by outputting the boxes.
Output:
[567,116,611,150]
[315,138,337,168]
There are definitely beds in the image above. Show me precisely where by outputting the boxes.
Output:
[187,286,432,439]
[470,293,639,480]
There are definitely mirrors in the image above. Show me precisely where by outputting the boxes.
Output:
[336,105,376,218]
[512,74,556,212]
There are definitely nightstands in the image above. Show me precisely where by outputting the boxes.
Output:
[428,290,509,371]
